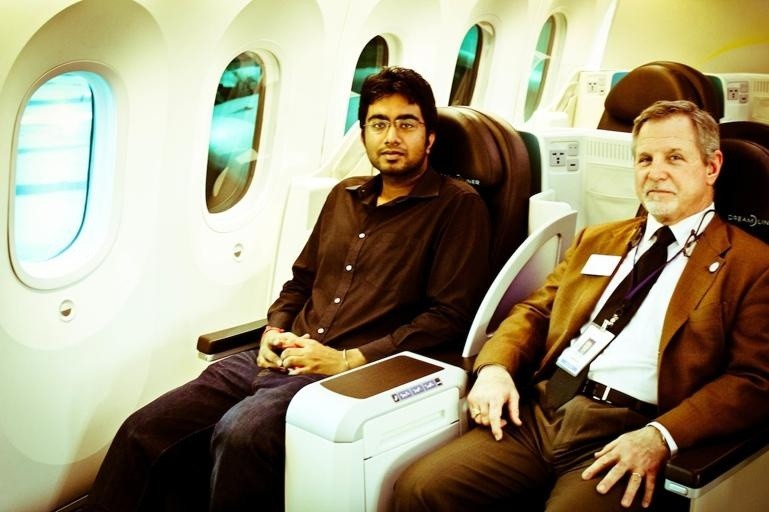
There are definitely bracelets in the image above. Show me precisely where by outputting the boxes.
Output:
[342,348,351,370]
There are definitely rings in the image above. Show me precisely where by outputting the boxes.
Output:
[470,406,481,417]
[631,465,644,480]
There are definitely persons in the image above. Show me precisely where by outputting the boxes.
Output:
[90,70,487,512]
[394,102,769,512]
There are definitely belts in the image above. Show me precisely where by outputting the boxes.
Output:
[581,379,659,418]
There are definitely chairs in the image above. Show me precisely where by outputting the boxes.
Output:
[198,103,532,410]
[592,119,767,511]
[598,55,717,131]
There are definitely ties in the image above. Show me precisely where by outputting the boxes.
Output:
[546,226,677,410]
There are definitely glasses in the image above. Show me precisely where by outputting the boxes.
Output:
[361,119,424,133]
